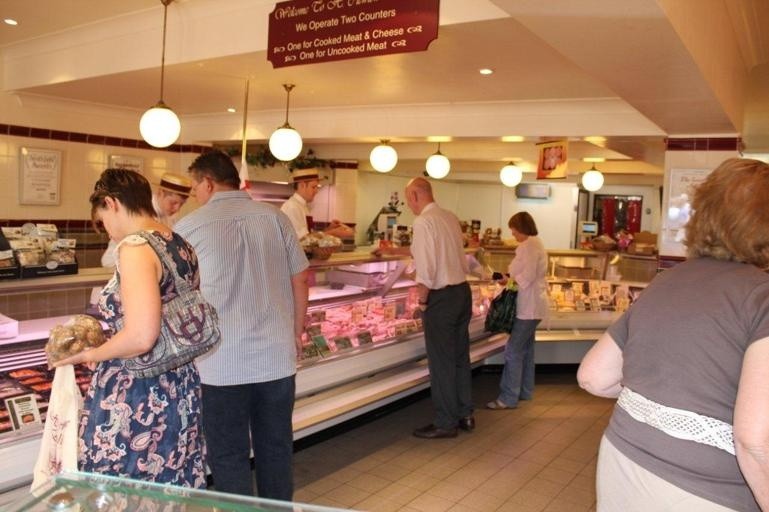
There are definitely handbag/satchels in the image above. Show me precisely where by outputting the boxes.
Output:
[116,230,221,378]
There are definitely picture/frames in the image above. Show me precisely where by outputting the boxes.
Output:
[18,146,62,205]
[107,154,144,176]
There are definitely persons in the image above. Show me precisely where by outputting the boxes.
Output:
[488,211,548,409]
[404,178,481,439]
[49,166,215,503]
[274,168,351,241]
[579,156,768,511]
[101,171,194,272]
[170,149,311,510]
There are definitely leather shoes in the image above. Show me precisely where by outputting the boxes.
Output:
[413,416,475,438]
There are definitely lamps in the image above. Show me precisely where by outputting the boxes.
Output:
[581,162,604,192]
[426,142,450,179]
[139,0,181,148]
[500,161,522,187]
[369,139,398,173]
[269,84,302,161]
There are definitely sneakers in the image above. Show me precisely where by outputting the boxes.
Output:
[485,278,518,334]
[487,399,509,409]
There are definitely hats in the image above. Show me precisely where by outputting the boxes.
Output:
[151,173,194,197]
[288,168,323,184]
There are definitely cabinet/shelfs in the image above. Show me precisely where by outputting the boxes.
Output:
[292,253,509,443]
[490,248,658,364]
